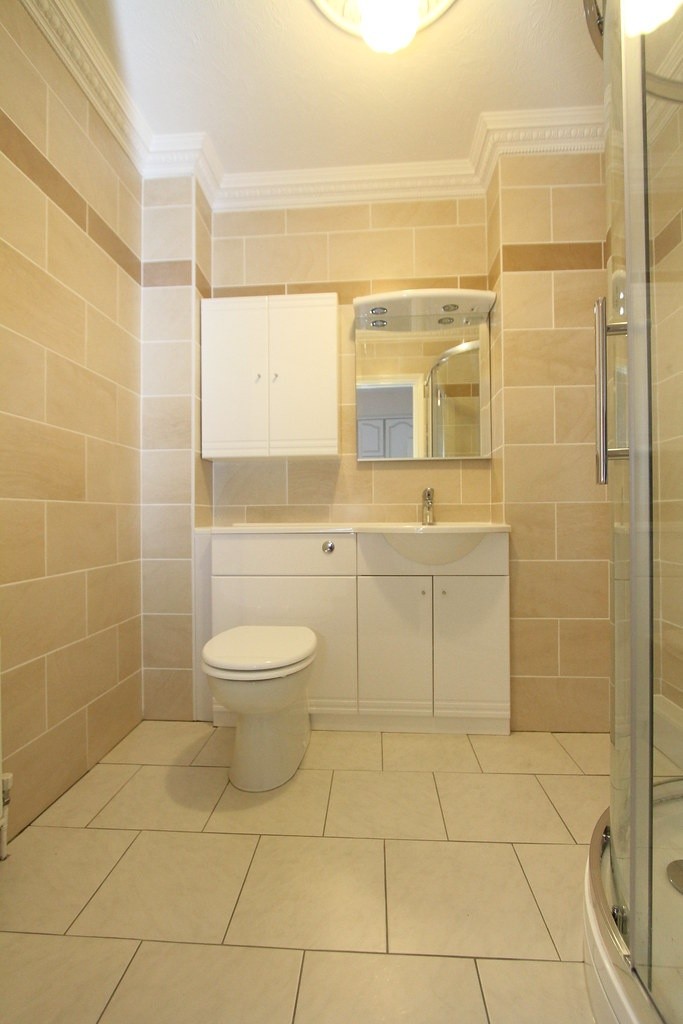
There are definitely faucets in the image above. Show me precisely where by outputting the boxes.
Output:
[422,487,435,525]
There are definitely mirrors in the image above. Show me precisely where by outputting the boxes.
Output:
[356,313,492,463]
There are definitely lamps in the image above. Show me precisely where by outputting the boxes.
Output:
[312,0,458,54]
[355,314,489,332]
[353,288,496,313]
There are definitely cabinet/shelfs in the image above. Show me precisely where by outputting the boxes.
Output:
[356,534,512,721]
[201,291,340,462]
[211,533,358,716]
[357,415,413,457]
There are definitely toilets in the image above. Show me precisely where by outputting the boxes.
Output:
[200,625,318,794]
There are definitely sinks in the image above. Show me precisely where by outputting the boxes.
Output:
[378,521,488,565]
